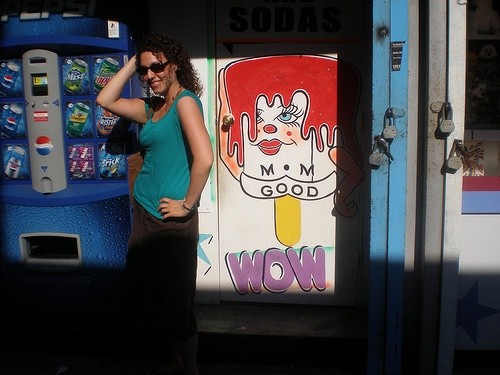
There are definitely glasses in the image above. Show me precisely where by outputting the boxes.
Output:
[136,60,171,76]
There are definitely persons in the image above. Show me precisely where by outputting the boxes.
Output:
[95,33,216,375]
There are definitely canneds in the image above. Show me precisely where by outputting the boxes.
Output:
[0,61,20,97]
[97,108,121,134]
[67,102,92,138]
[101,145,122,175]
[4,145,28,179]
[70,146,94,178]
[94,58,120,94]
[63,57,89,94]
[2,103,24,138]
